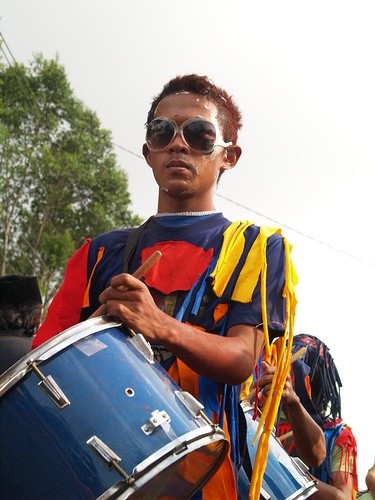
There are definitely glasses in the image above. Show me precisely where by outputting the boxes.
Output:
[146,116,233,155]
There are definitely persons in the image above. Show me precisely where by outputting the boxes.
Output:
[0,275,42,376]
[245,333,375,500]
[32,75,299,500]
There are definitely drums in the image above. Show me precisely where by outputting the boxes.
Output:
[0,313,230,500]
[213,396,320,500]
